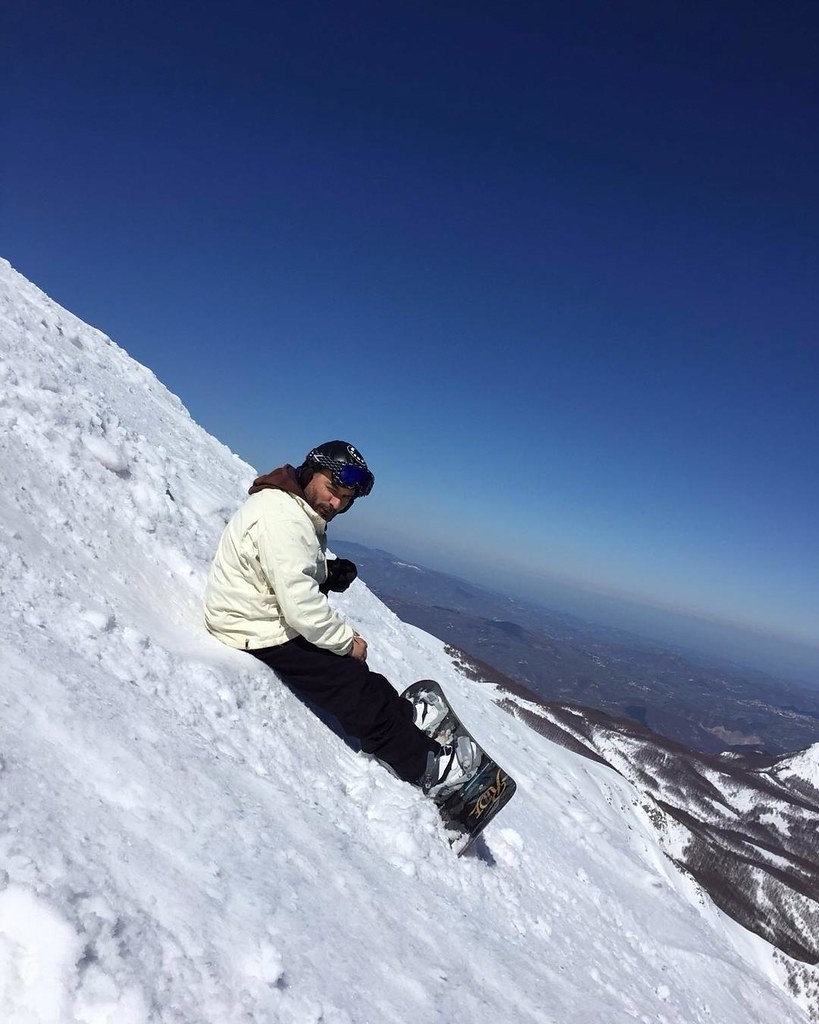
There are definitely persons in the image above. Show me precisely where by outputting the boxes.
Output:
[204,441,478,797]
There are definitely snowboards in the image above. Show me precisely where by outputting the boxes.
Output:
[397,677,519,862]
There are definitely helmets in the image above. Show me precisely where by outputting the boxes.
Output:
[302,440,374,514]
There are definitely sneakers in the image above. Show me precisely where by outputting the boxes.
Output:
[414,696,442,730]
[428,742,476,804]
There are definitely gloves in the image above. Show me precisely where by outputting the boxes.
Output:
[316,558,358,600]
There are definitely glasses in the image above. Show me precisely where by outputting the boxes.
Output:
[331,461,375,496]
